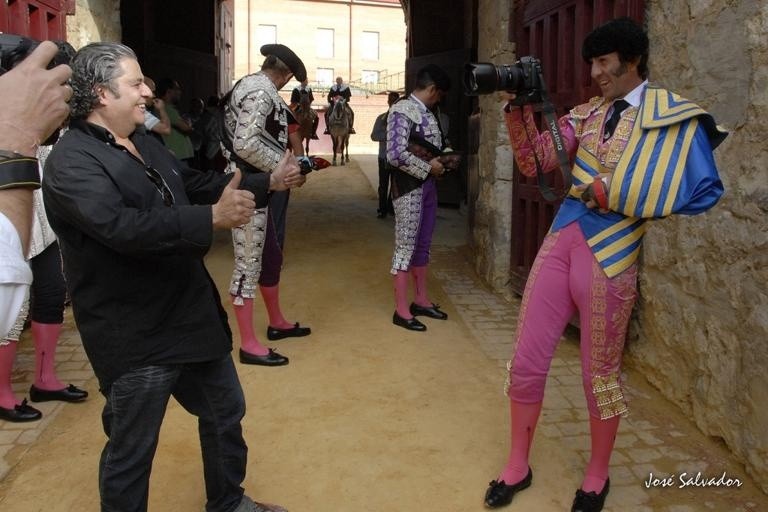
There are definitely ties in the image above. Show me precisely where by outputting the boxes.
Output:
[603,100,630,143]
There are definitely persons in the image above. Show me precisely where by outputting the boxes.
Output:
[385,63,464,331]
[291,77,319,140]
[217,43,315,366]
[41,42,292,512]
[143,77,208,172]
[482,17,730,512]
[323,76,357,134]
[370,92,400,218]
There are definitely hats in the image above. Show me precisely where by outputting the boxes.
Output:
[260,44,307,82]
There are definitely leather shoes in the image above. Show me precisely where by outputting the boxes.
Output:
[484,465,533,508]
[570,477,609,512]
[393,310,426,331]
[377,212,386,218]
[267,322,311,340]
[410,302,447,320]
[0,397,41,422]
[239,348,289,366]
[30,383,88,402]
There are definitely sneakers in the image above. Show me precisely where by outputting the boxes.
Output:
[232,494,289,512]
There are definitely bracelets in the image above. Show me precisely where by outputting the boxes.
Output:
[0,149,41,190]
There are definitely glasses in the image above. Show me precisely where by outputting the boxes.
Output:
[146,166,173,206]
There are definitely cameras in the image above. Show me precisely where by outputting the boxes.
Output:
[0,30,75,145]
[468,54,540,108]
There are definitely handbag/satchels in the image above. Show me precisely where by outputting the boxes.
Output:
[201,106,226,142]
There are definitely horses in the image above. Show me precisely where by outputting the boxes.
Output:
[329,96,350,166]
[294,90,312,157]
